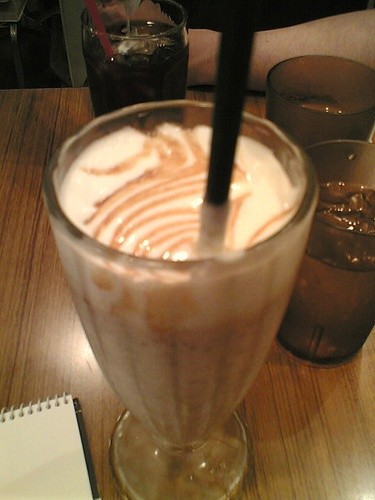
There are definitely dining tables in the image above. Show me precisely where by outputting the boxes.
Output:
[0,88,375,500]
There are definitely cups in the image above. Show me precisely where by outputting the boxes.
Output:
[276,139,375,364]
[42,100,318,499]
[265,55,375,149]
[82,0,189,117]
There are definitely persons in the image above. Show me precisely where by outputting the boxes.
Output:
[185,9,375,93]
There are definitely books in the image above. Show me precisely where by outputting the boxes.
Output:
[0,392,101,500]
